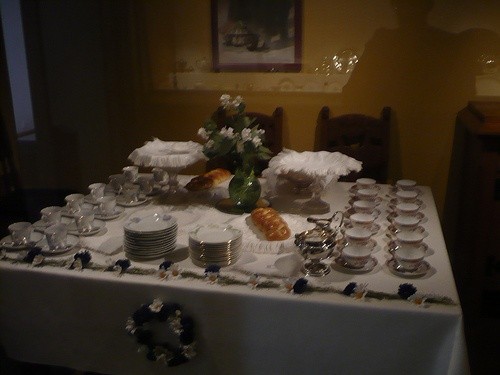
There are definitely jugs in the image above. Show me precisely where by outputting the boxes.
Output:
[296,210,344,277]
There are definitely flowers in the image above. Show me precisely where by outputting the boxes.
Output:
[198,93,264,151]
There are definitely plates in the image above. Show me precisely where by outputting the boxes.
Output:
[189,223,244,268]
[0,174,178,259]
[334,183,431,277]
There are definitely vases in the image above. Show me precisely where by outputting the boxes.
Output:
[228,164,261,210]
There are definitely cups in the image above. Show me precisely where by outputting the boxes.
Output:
[394,247,424,271]
[340,228,372,247]
[7,165,170,249]
[348,200,376,214]
[344,213,375,230]
[335,246,371,269]
[396,180,417,192]
[395,231,423,250]
[395,216,419,233]
[396,203,419,218]
[351,178,376,190]
[352,188,377,202]
[396,190,418,205]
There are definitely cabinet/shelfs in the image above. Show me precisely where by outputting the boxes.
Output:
[444,109,500,308]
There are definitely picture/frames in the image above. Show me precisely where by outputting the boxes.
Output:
[210,0,302,74]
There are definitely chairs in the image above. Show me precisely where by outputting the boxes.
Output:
[314,105,390,182]
[209,107,283,157]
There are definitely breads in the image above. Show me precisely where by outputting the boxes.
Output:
[251,207,292,241]
[182,169,231,190]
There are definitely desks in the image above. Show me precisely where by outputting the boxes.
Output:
[0,171,463,375]
